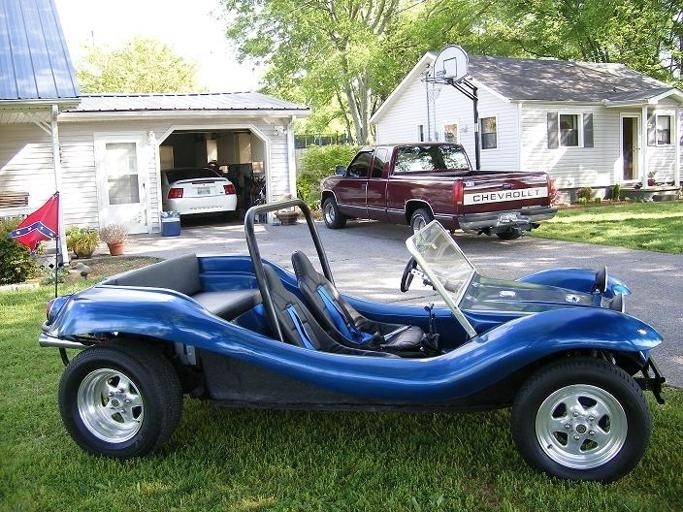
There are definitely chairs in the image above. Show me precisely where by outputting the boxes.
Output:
[291,250,423,351]
[260,263,402,359]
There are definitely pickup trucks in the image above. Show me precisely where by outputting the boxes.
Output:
[320,142,559,240]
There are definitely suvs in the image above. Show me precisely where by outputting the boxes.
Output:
[37,199,666,484]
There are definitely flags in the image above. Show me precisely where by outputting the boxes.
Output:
[6,191,59,256]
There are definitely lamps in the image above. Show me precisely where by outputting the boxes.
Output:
[273,126,283,136]
[647,122,652,128]
[147,131,155,145]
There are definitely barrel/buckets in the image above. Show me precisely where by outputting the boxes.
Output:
[162,218,181,237]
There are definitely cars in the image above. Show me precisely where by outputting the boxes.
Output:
[161,167,238,227]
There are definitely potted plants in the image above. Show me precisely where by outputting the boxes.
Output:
[648,171,657,186]
[65,225,99,258]
[100,223,129,255]
